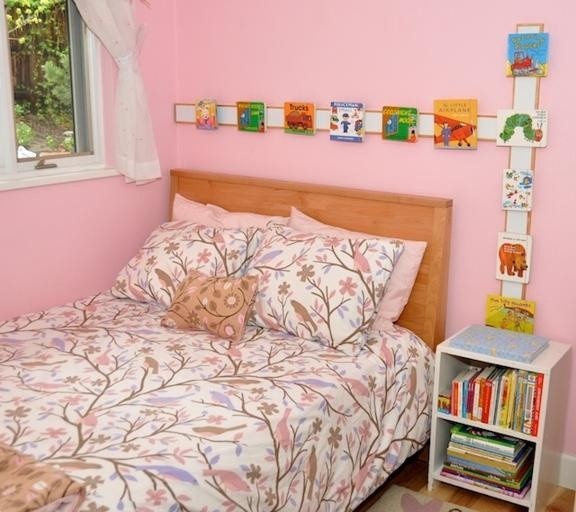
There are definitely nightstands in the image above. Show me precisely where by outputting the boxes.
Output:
[425,319,576,512]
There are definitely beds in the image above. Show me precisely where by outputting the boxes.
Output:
[0,167,457,511]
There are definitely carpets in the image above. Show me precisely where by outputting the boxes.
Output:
[364,480,476,512]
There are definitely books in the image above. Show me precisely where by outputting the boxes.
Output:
[446,322,550,365]
[436,359,545,501]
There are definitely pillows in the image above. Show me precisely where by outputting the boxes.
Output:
[289,204,428,331]
[170,192,288,233]
[109,221,261,307]
[160,270,259,342]
[243,218,406,358]
